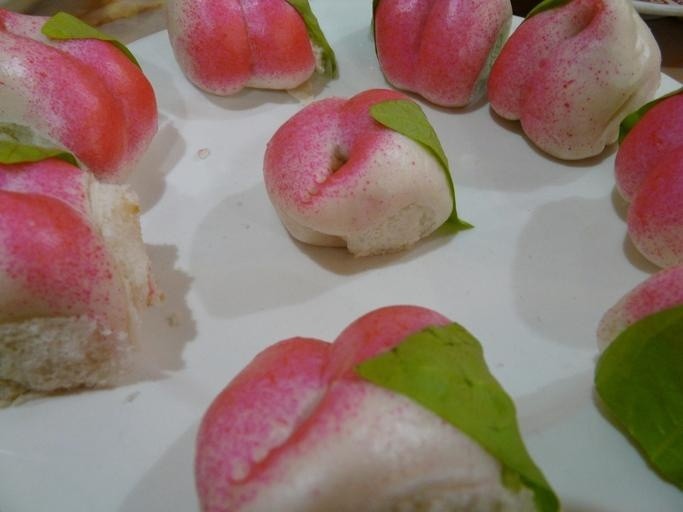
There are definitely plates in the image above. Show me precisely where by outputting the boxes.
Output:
[3,2,682,509]
